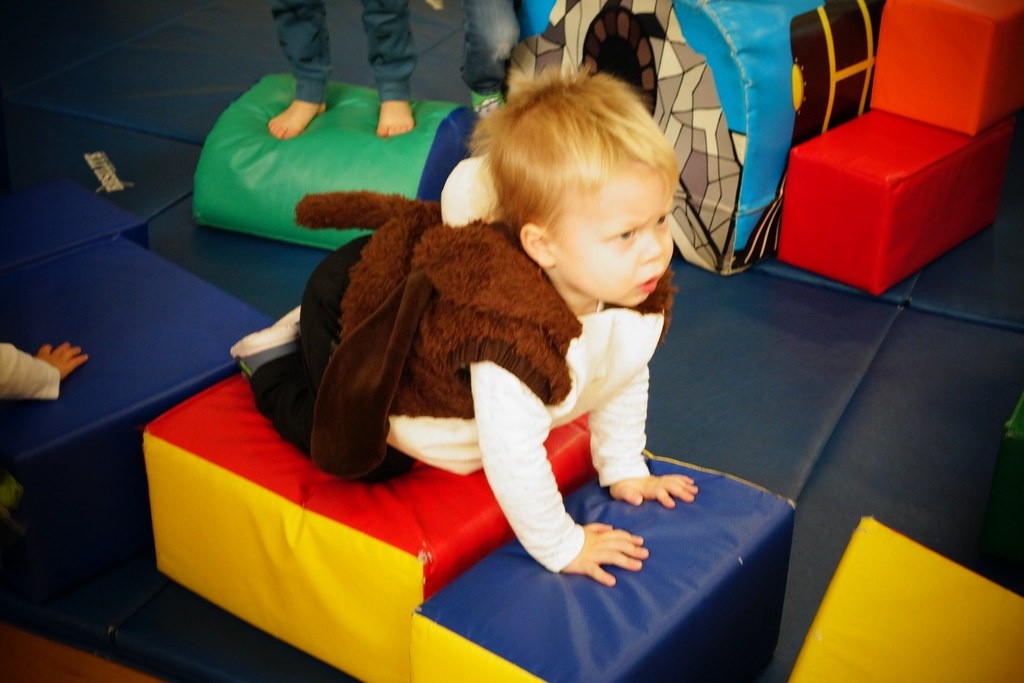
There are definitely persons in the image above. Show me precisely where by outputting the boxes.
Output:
[266,0,418,141]
[459,0,522,116]
[229,64,697,588]
[0,341,87,400]
